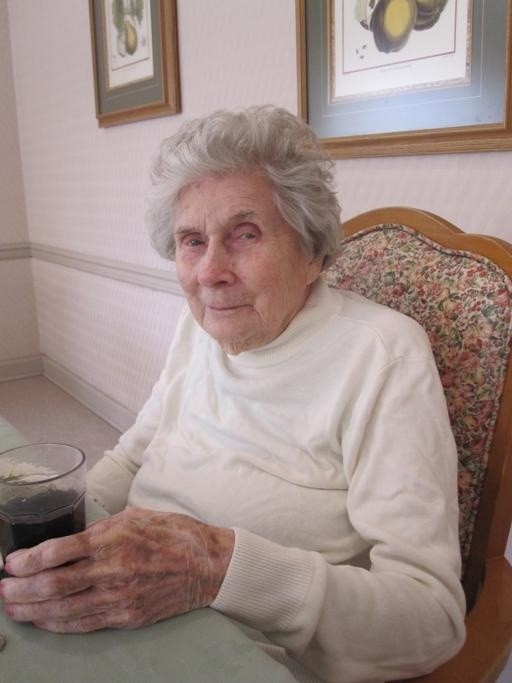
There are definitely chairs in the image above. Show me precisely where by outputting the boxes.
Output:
[322,208,512,680]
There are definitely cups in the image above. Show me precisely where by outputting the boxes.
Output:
[0,441,89,577]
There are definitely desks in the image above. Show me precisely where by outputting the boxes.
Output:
[2,426,321,682]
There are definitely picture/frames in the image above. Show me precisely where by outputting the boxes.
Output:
[296,2,510,159]
[88,1,182,129]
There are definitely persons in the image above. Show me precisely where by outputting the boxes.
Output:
[0,101,469,683]
